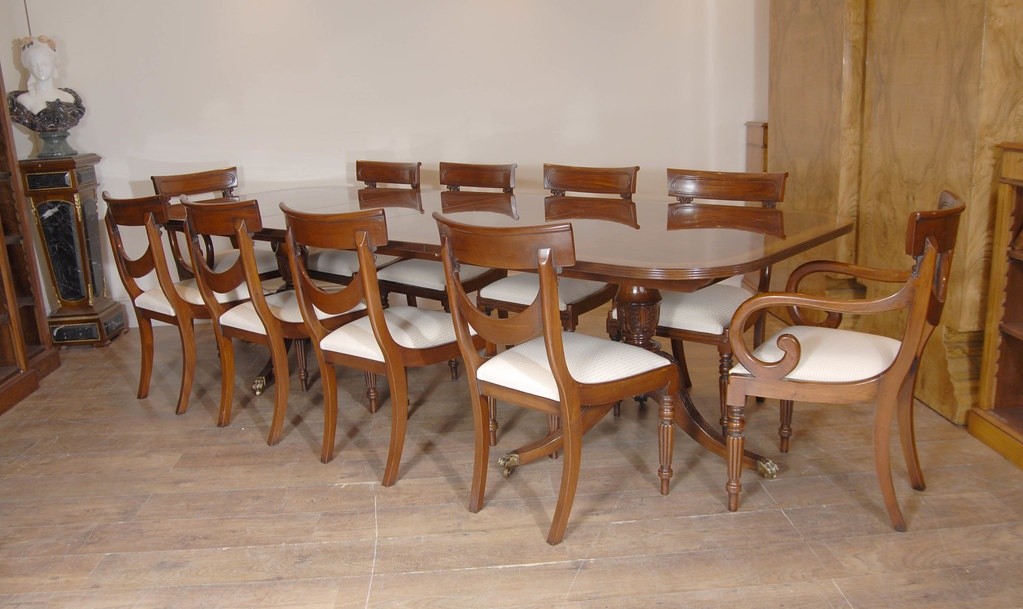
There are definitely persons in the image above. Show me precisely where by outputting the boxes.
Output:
[20,37,75,113]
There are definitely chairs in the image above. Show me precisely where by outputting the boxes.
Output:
[377,162,519,379]
[179,194,378,447]
[151,167,282,358]
[432,212,678,546]
[607,169,790,438]
[478,163,641,332]
[305,160,424,307]
[280,201,498,487]
[102,190,274,415]
[726,190,967,530]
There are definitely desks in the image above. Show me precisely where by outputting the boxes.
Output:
[164,186,853,479]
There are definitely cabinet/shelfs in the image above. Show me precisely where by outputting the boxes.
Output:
[0,65,61,415]
[967,142,1023,471]
